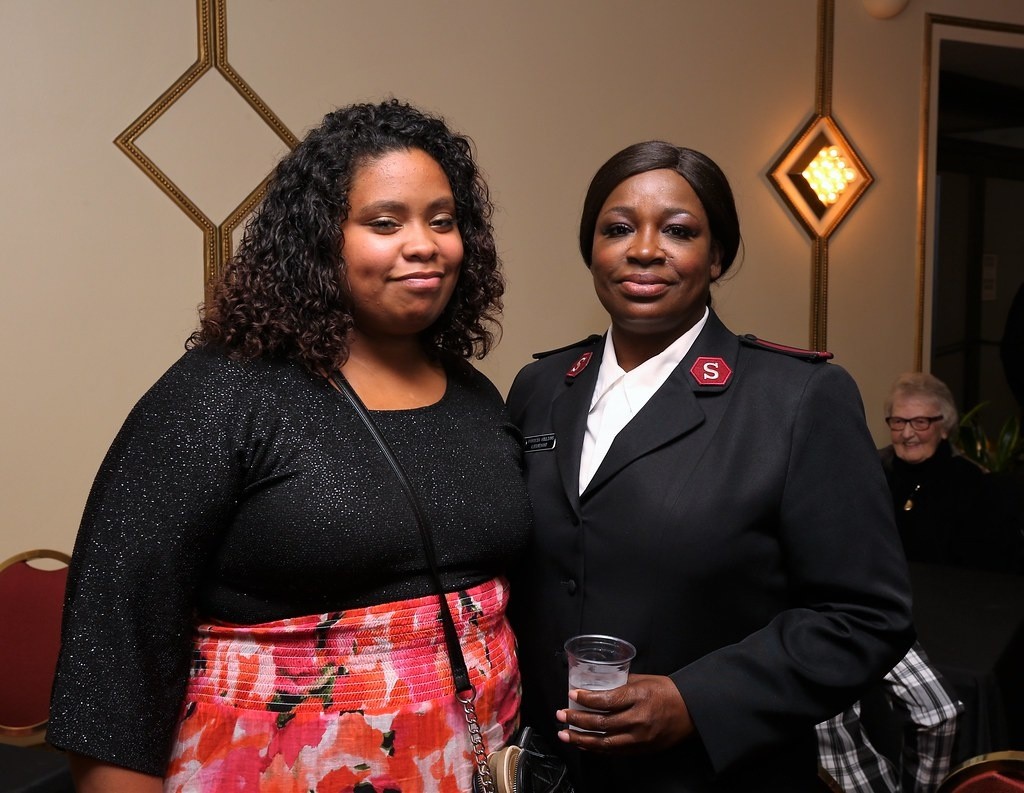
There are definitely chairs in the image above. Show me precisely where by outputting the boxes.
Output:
[0,549,70,793]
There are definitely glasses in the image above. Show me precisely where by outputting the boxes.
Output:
[885,415,944,431]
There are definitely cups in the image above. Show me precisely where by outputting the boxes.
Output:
[563,633,637,736]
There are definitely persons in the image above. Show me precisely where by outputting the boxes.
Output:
[506,139,917,792]
[43,97,536,793]
[876,371,1024,578]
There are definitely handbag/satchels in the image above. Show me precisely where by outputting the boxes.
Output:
[472,745,574,793]
